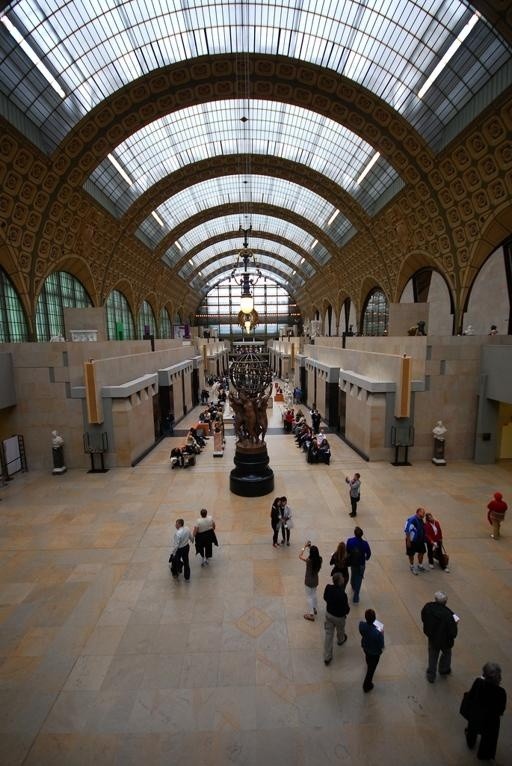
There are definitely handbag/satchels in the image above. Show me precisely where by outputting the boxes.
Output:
[460,693,482,725]
[353,493,360,502]
[443,553,449,566]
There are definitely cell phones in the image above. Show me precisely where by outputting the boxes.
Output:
[308,541,311,546]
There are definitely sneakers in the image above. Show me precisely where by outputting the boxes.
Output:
[410,564,449,575]
[304,614,315,621]
[337,634,347,646]
[325,658,332,665]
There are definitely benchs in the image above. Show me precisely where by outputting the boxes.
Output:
[178,396,223,467]
[273,383,330,463]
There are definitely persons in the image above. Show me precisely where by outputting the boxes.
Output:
[487,492,507,540]
[276,386,330,466]
[464,661,508,764]
[171,509,218,583]
[346,472,361,517]
[52,430,65,469]
[359,608,384,693]
[299,526,371,667]
[404,508,449,576]
[420,591,457,684]
[168,367,230,469]
[271,496,293,548]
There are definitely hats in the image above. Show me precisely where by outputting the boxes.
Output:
[495,492,502,501]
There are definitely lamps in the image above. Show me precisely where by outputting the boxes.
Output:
[230,210,262,335]
